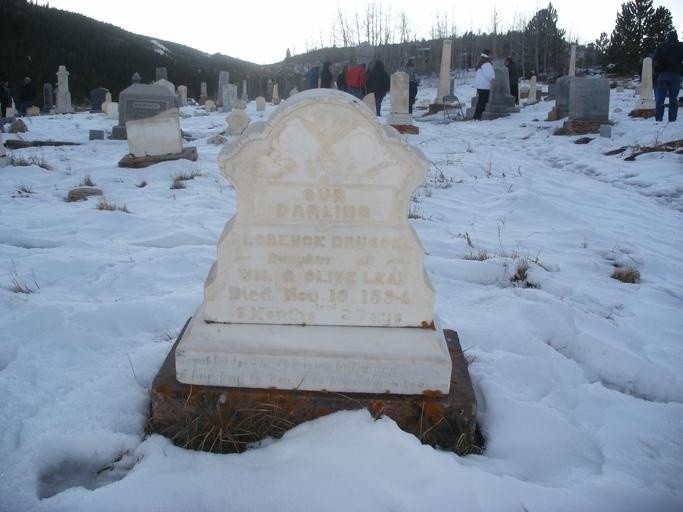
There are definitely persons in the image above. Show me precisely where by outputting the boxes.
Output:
[652,30,683,122]
[471,49,497,121]
[504,56,520,105]
[278,57,422,116]
